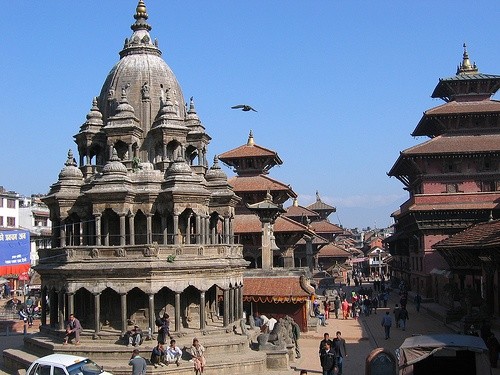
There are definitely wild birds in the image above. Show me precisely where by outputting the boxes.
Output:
[231,104,258,113]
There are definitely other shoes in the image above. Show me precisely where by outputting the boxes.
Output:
[166,361,168,366]
[154,363,158,367]
[128,344,132,347]
[135,344,139,346]
[159,362,164,366]
[63,341,67,344]
[75,341,79,345]
[176,362,180,364]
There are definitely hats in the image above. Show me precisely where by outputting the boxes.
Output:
[193,338,198,343]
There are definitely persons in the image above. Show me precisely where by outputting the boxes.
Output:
[0,269,500,375]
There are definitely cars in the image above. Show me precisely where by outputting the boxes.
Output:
[26,354,115,375]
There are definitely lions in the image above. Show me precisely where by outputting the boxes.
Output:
[257,315,294,346]
